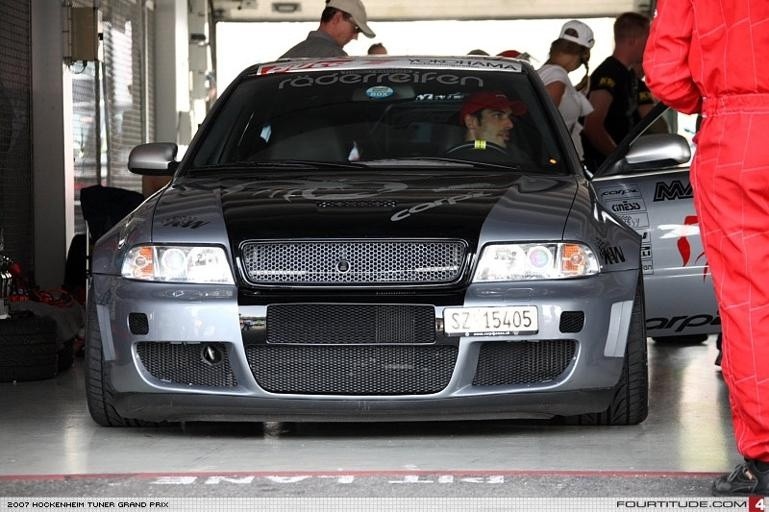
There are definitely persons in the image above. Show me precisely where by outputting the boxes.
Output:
[277,0,377,60]
[457,90,529,158]
[534,9,670,177]
[642,0,768,500]
[368,41,388,56]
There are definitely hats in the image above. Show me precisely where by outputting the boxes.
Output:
[558,20,595,49]
[461,91,529,119]
[325,1,376,38]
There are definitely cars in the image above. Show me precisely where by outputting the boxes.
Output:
[84,53,723,430]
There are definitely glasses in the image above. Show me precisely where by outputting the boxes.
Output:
[344,15,361,33]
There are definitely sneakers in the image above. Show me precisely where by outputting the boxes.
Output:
[711,460,769,498]
